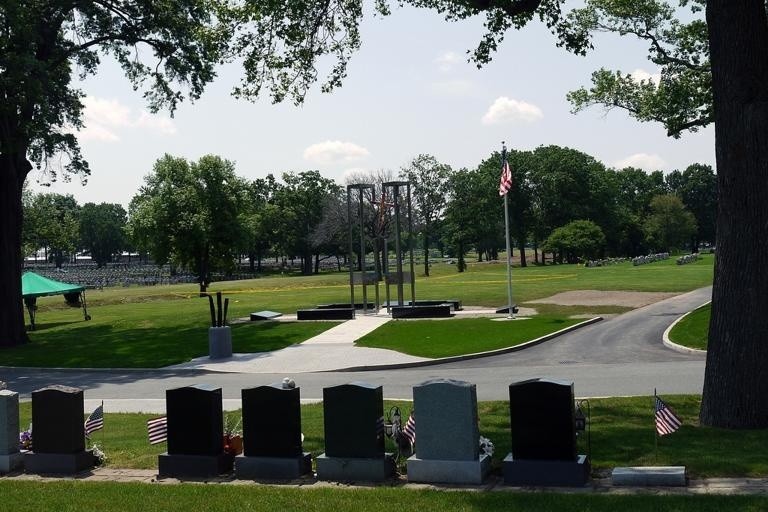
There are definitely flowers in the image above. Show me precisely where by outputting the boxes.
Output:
[18,422,33,450]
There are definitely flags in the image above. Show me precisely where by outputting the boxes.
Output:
[146,415,168,446]
[655,396,683,438]
[403,409,416,448]
[498,145,513,197]
[84,405,104,436]
